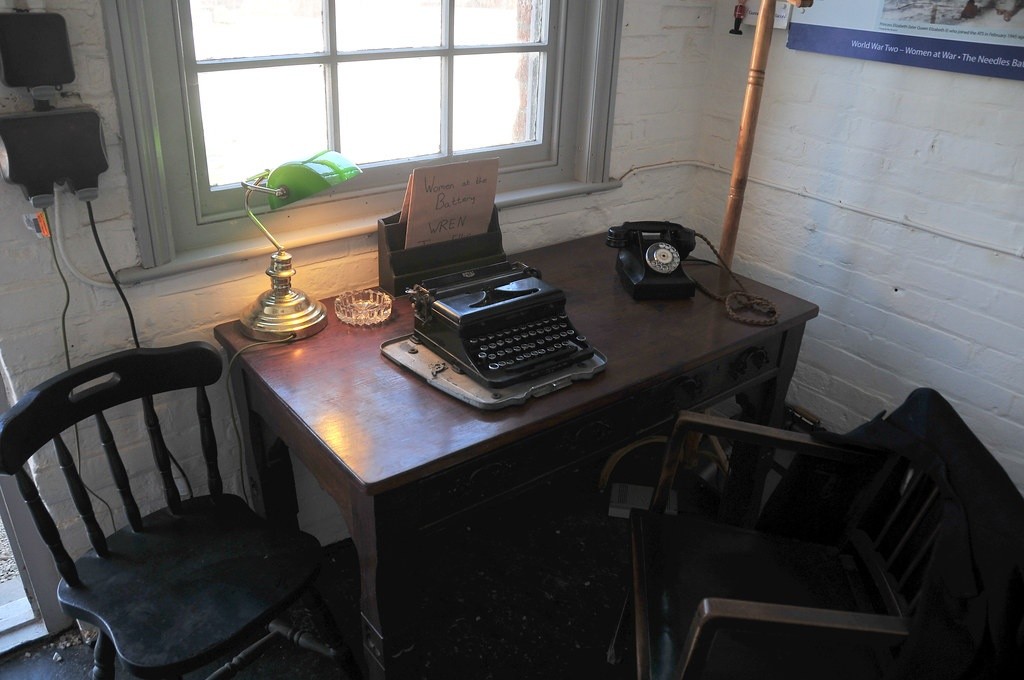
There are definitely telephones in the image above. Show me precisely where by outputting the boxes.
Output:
[602,218,699,298]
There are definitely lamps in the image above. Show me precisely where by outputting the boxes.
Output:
[237,149,364,345]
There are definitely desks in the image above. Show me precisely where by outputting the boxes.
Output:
[214,230,819,680]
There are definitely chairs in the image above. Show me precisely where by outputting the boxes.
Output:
[0,341,341,680]
[599,388,1024,680]
